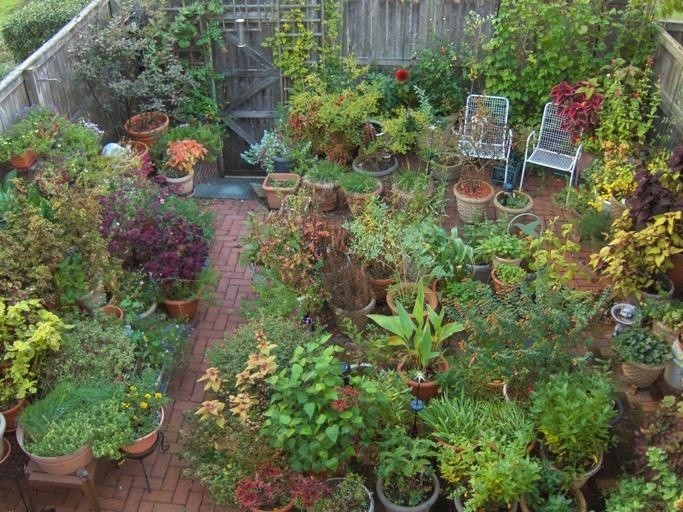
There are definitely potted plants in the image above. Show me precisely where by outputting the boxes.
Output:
[1,1,682,512]
